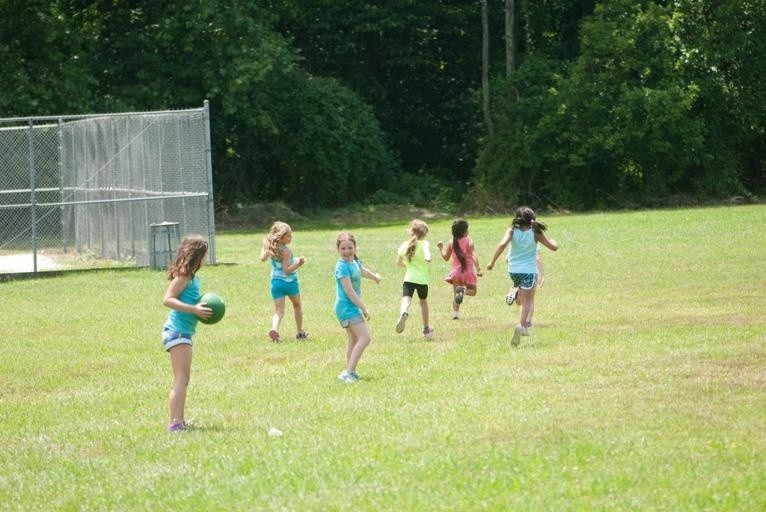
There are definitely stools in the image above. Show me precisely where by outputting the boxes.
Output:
[152,222,181,265]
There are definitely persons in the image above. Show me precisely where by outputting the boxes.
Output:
[261,221,310,342]
[437,220,483,319]
[162,236,208,433]
[487,206,558,347]
[395,220,434,338]
[522,248,544,334]
[335,231,382,383]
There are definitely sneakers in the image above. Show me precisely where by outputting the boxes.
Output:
[396,312,409,332]
[338,370,359,383]
[506,287,518,304]
[455,286,465,303]
[269,330,281,343]
[511,322,531,344]
[297,331,310,339]
[170,420,187,432]
[454,311,460,319]
[423,326,434,337]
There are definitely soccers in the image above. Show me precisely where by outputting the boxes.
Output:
[195,293,224,325]
